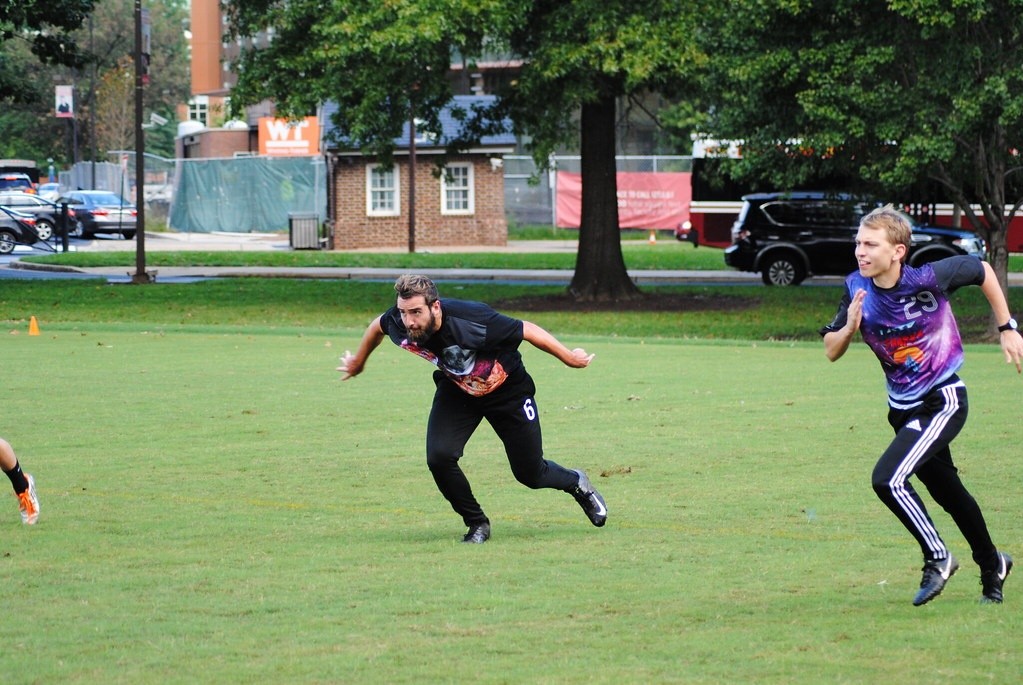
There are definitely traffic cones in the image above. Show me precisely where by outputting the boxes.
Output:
[28,316,40,335]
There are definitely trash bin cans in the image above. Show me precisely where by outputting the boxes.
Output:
[288,211,319,250]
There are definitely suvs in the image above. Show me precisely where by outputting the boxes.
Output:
[723,190,988,286]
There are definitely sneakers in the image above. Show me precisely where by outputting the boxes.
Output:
[913,553,959,606]
[15,475,39,525]
[569,469,608,527]
[463,522,491,545]
[978,553,1013,607]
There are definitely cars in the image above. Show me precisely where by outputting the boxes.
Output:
[0,207,42,256]
[52,190,138,240]
[0,171,60,203]
[0,193,79,239]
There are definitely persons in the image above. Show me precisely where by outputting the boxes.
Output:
[819,207,1023,606]
[0,438,40,525]
[337,273,608,545]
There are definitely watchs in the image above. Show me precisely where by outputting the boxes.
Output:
[998,318,1018,332]
